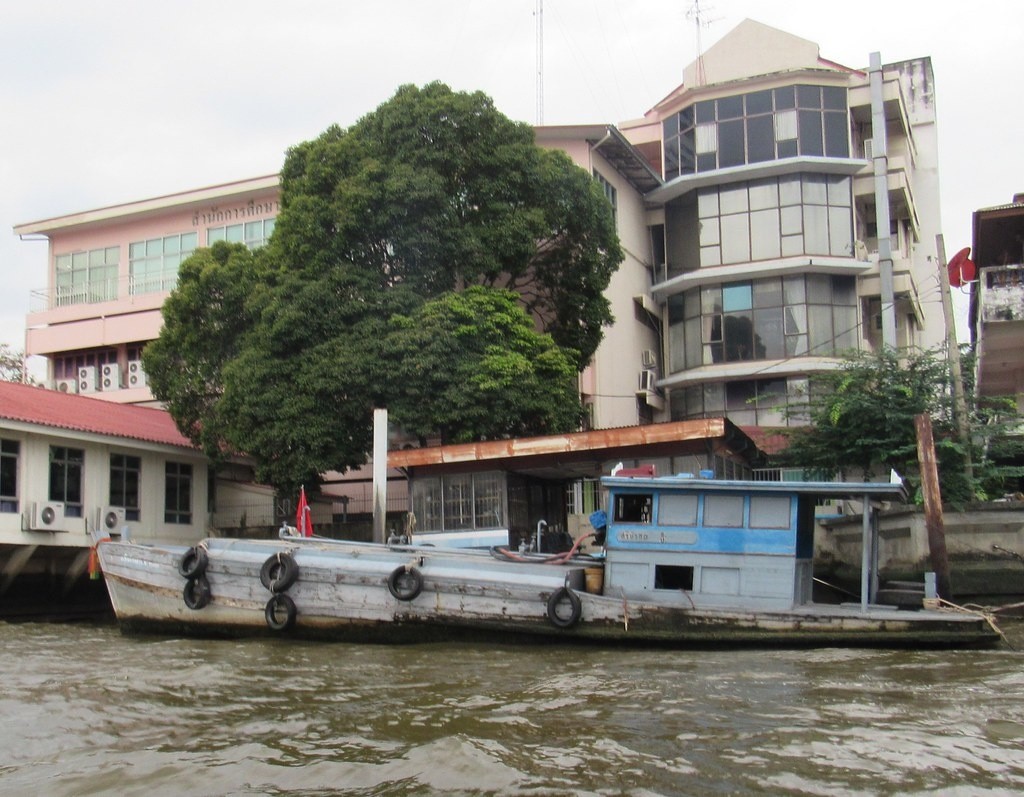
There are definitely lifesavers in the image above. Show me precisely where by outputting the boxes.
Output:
[547,587,582,626]
[265,594,297,630]
[177,548,206,577]
[387,565,423,600]
[260,551,299,591]
[183,576,211,608]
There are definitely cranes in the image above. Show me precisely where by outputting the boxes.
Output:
[524,0,549,124]
[687,1,728,86]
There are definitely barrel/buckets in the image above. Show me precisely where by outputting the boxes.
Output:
[584,568,603,594]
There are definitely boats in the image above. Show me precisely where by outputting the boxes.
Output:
[95,464,1005,651]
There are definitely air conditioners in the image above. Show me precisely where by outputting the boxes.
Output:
[25,499,65,529]
[56,379,78,393]
[863,138,873,161]
[128,375,146,388]
[102,363,119,377]
[128,360,145,375]
[78,366,99,380]
[79,380,99,394]
[102,377,119,392]
[36,380,56,391]
[93,504,126,534]
[639,370,657,393]
[643,349,657,368]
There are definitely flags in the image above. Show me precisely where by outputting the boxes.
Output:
[296,489,312,537]
